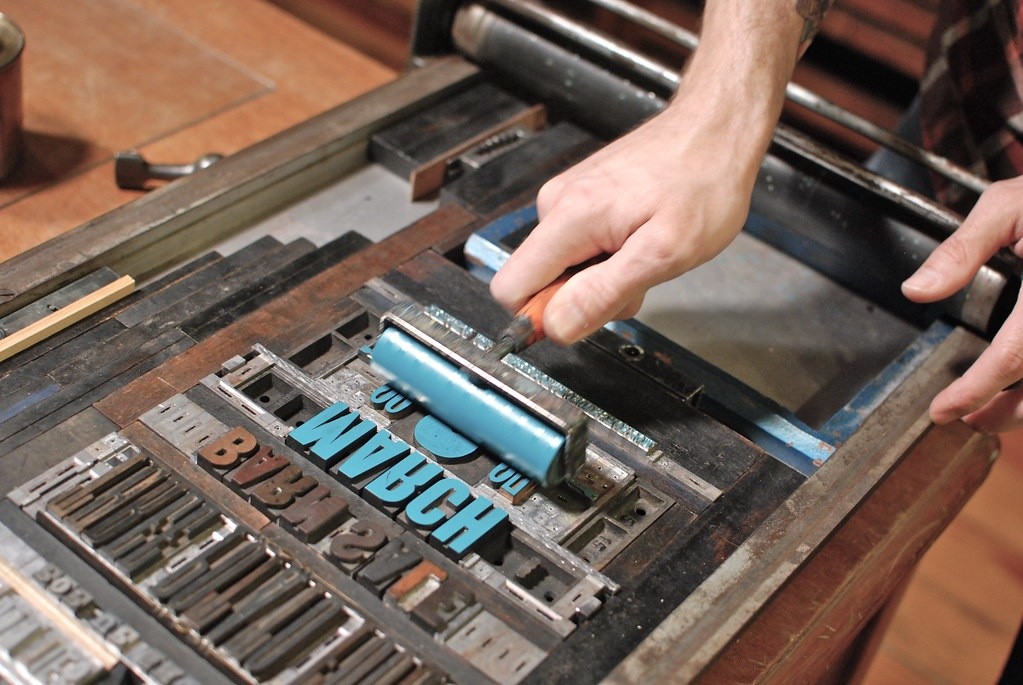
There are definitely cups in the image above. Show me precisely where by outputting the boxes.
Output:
[0,10,26,186]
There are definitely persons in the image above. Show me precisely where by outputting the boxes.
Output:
[488,0,1023,434]
